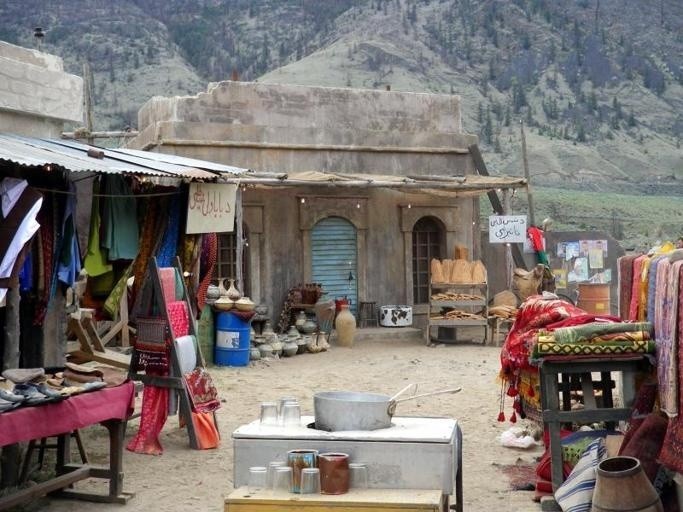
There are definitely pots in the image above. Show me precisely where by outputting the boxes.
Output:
[316,388,464,434]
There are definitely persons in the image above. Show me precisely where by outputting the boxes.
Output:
[558,239,607,282]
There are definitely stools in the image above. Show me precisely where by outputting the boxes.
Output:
[489,317,514,346]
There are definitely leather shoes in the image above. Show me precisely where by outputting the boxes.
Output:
[0,377,108,413]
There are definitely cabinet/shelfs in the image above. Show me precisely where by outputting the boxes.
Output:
[427,281,490,346]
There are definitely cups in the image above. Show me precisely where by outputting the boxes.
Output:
[280,398,301,429]
[248,467,268,494]
[299,466,322,498]
[261,403,279,426]
[275,468,293,497]
[347,461,370,493]
[267,462,287,492]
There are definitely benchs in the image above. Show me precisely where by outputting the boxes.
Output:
[222,482,448,509]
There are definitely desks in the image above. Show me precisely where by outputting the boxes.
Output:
[532,357,654,496]
[0,382,135,509]
[232,414,463,511]
